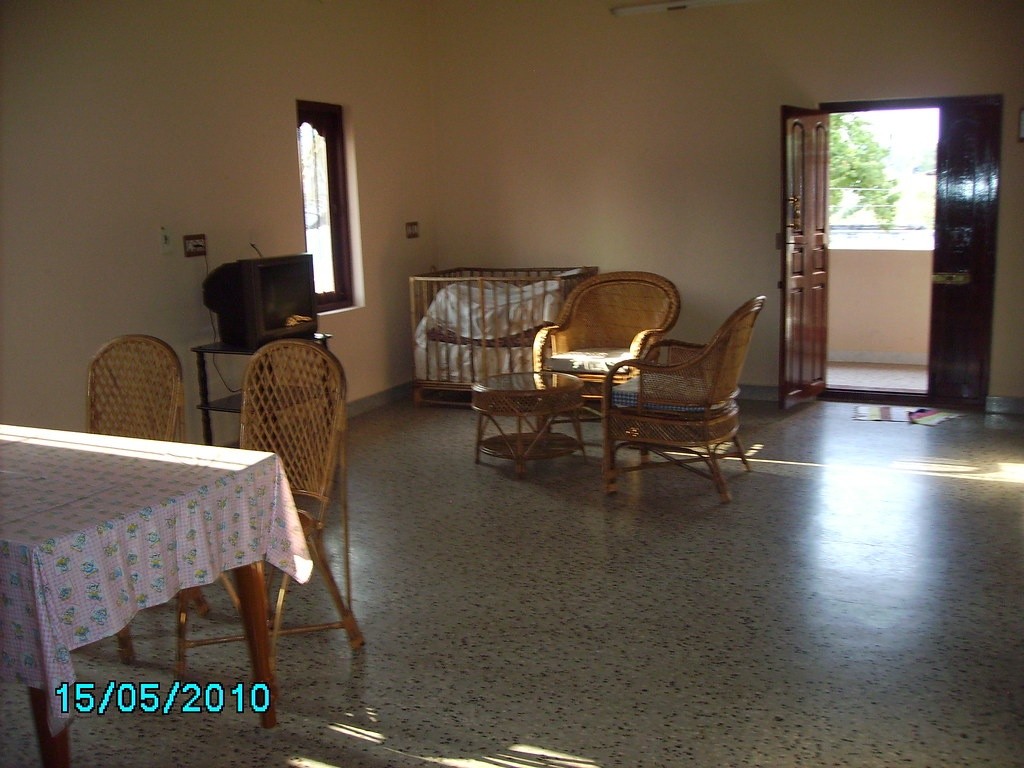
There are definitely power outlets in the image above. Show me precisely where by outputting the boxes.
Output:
[187,237,206,256]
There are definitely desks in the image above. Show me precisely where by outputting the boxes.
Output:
[471,371,590,481]
[0,423,314,768]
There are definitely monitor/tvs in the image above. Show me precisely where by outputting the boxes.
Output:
[202,253,318,351]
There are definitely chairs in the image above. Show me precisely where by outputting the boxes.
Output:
[83,334,366,680]
[533,271,767,503]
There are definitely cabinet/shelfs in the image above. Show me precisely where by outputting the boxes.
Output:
[191,335,331,445]
